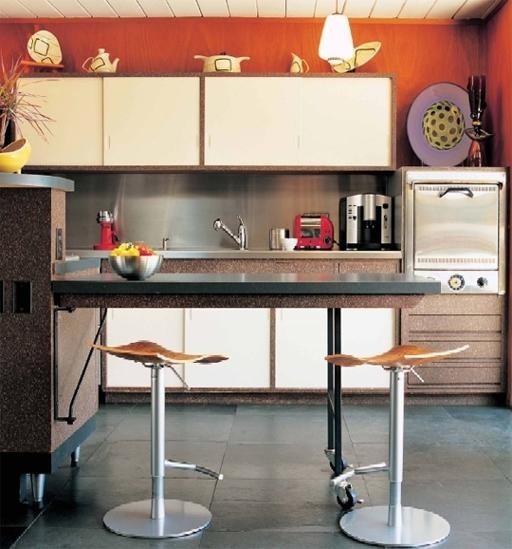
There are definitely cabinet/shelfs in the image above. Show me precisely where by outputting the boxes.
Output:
[12,70,200,172]
[270,308,399,394]
[101,307,270,392]
[201,73,398,172]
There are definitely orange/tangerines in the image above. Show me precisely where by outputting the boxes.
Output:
[110,243,140,257]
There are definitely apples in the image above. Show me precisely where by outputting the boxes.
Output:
[138,245,153,256]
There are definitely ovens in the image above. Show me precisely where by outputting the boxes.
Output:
[401,167,508,294]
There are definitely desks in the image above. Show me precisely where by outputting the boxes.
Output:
[52,272,440,509]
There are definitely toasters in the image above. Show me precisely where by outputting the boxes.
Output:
[293,211,335,250]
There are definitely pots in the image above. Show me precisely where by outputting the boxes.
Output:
[192,52,252,73]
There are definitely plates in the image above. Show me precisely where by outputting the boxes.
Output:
[406,81,472,167]
[26,29,63,65]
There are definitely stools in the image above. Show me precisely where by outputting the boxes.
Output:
[93,339,228,540]
[325,346,469,547]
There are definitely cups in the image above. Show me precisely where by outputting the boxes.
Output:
[270,228,290,250]
[279,237,299,251]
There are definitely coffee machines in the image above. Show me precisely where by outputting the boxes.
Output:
[338,192,396,251]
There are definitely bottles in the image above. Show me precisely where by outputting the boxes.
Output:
[465,74,486,135]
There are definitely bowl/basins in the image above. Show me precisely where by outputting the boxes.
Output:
[108,255,165,280]
[0,137,36,173]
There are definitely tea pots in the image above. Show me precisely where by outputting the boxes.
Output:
[82,48,121,74]
[287,50,310,74]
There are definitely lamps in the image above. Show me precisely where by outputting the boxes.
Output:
[318,0,354,65]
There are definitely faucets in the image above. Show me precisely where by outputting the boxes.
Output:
[212,214,249,250]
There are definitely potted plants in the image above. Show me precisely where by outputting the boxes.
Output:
[0,50,55,173]
